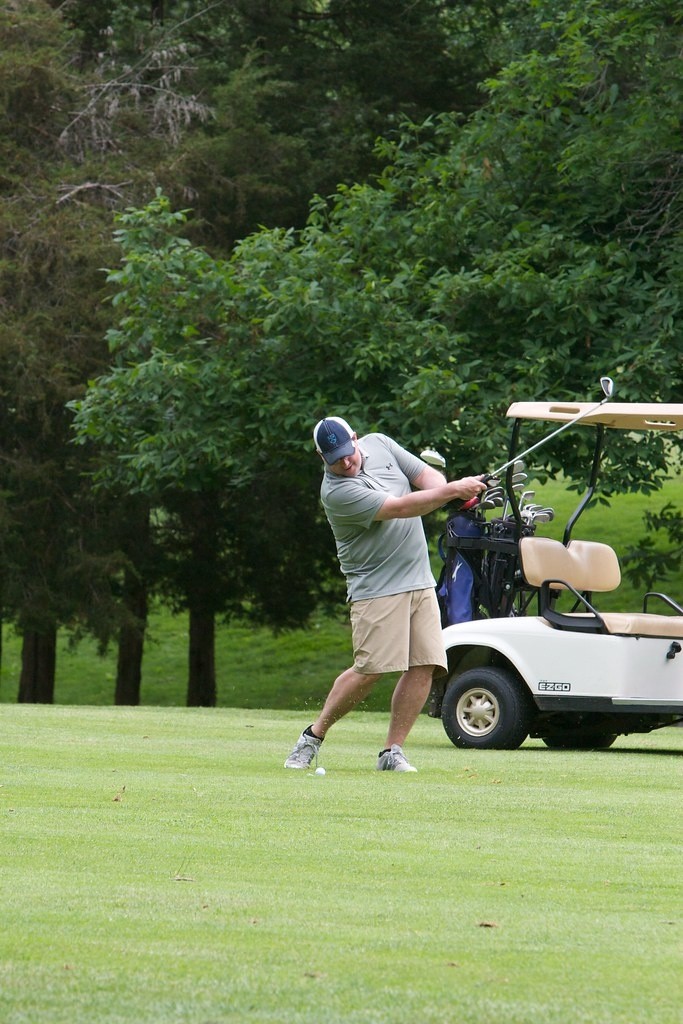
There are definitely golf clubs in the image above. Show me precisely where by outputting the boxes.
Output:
[474,500,496,519]
[480,486,505,519]
[518,490,536,512]
[439,376,615,513]
[520,503,554,526]
[502,459,528,522]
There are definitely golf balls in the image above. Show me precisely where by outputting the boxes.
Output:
[314,767,326,776]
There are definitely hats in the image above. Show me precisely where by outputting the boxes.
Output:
[313,417,356,465]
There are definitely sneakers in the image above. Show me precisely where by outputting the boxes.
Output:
[284,724,324,770]
[377,744,416,773]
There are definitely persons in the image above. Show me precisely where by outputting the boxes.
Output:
[283,416,486,773]
